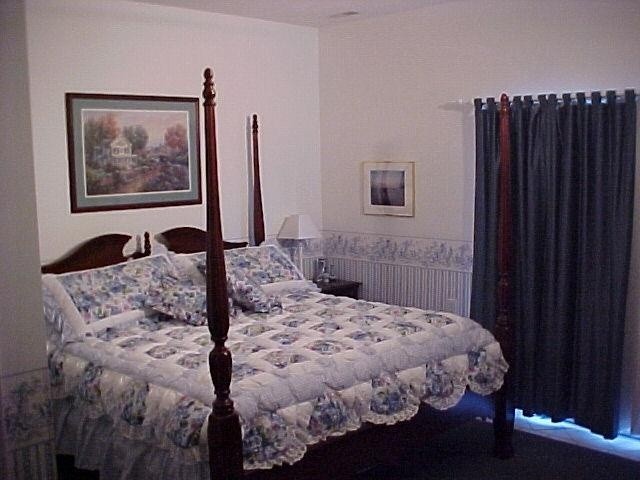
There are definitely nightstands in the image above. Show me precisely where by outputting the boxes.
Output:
[310,277,363,300]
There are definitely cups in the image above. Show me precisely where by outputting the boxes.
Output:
[313,272,323,281]
[322,273,329,282]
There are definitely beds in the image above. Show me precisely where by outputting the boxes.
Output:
[41,226,512,479]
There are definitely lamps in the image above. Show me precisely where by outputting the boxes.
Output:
[278,214,322,275]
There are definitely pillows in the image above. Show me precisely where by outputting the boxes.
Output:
[41,253,181,346]
[169,238,322,313]
[145,285,241,327]
[198,257,282,311]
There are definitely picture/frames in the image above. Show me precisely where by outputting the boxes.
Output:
[64,91,204,214]
[362,161,416,217]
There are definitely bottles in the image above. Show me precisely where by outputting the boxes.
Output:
[317,258,326,273]
